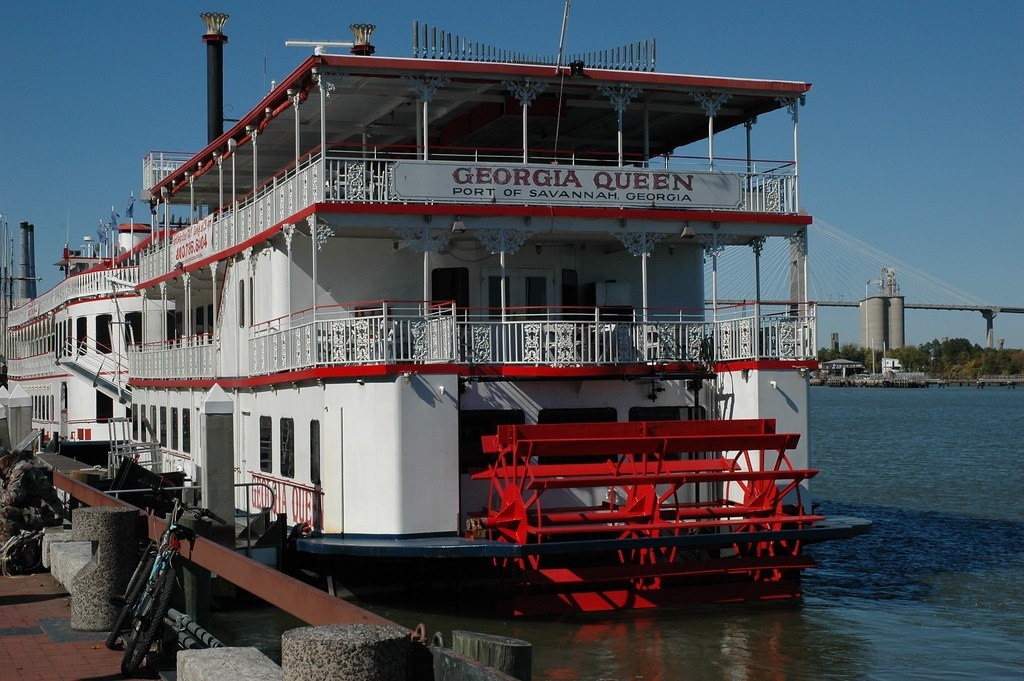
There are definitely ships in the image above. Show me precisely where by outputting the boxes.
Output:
[11,9,830,625]
